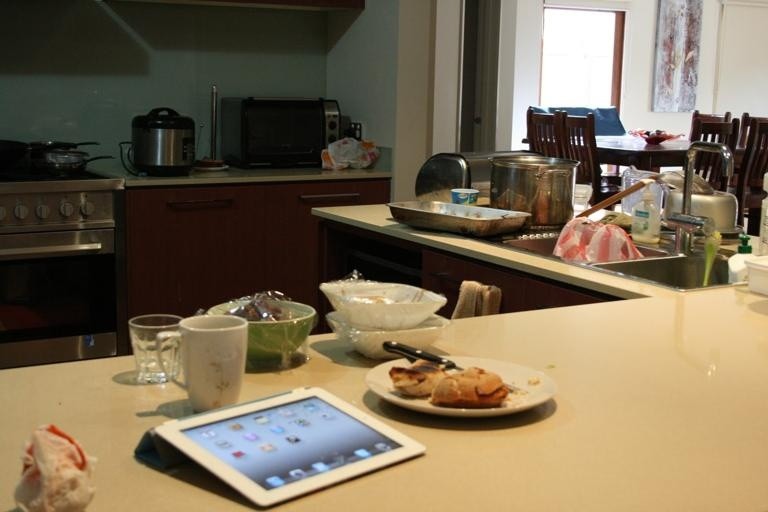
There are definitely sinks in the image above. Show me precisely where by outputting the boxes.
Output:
[589,254,730,291]
[498,230,671,264]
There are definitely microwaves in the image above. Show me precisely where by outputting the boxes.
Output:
[219,95,343,169]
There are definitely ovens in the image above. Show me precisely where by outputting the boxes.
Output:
[1,229,128,373]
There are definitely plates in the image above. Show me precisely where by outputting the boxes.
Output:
[364,353,559,419]
[195,164,231,172]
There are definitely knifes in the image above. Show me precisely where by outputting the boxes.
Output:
[384,342,518,394]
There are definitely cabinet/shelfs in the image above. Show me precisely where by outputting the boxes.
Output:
[262,173,397,332]
[120,185,265,348]
[420,240,529,321]
[521,275,621,310]
[330,219,422,313]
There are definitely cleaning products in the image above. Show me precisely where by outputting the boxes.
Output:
[737,234,753,253]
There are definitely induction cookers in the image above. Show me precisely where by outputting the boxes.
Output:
[1,174,124,234]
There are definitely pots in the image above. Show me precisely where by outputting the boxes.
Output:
[485,154,581,226]
[1,138,118,175]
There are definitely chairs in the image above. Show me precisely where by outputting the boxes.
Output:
[688,116,741,196]
[559,108,624,210]
[689,109,732,141]
[734,110,767,237]
[524,103,564,163]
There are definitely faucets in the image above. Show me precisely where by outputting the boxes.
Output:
[683,142,732,215]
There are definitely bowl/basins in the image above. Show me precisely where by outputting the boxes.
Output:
[627,129,686,145]
[207,279,449,357]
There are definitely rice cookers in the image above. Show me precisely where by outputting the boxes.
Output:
[130,106,197,177]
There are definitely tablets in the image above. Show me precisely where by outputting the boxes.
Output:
[154,386,426,509]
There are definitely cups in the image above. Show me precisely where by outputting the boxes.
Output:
[449,188,481,206]
[620,167,671,220]
[128,312,249,415]
[572,182,592,215]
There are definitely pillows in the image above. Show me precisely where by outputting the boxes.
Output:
[549,104,627,137]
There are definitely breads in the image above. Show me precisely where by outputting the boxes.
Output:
[389,359,446,398]
[431,368,510,410]
